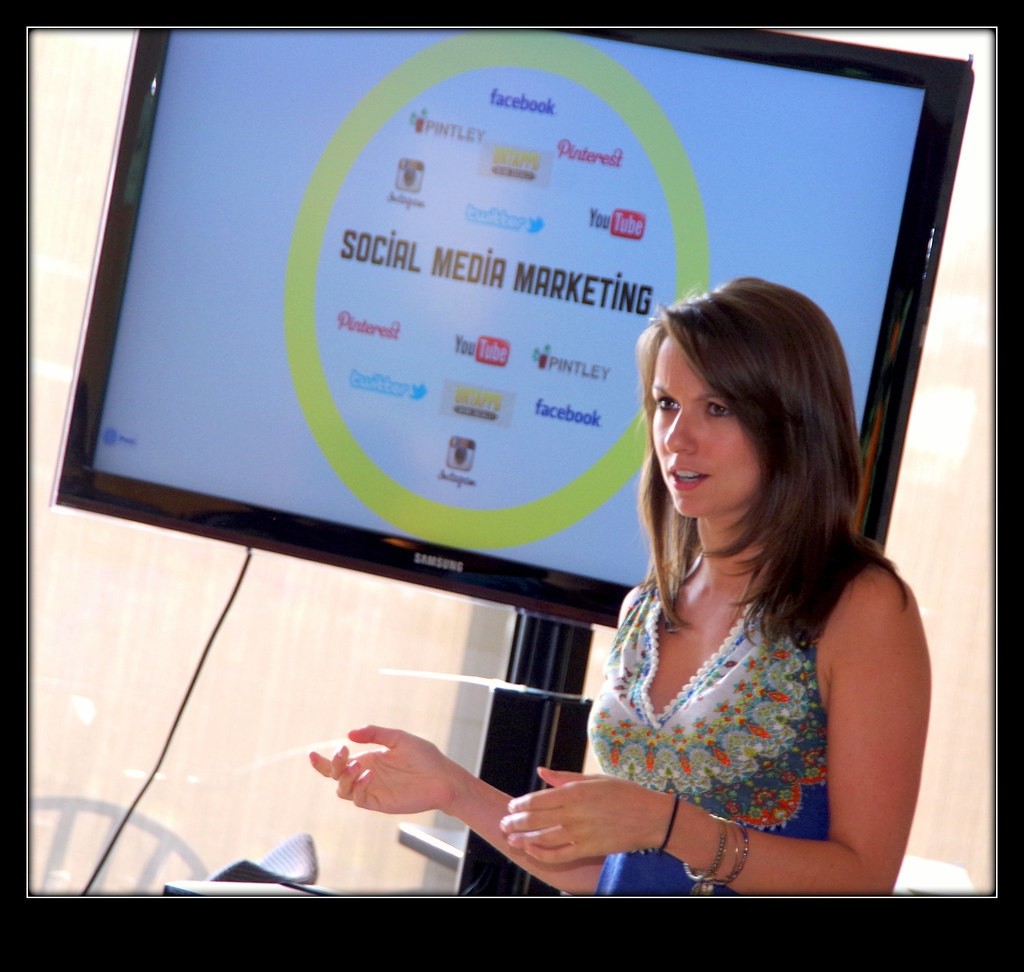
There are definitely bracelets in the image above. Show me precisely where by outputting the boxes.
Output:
[659,792,680,855]
[683,813,749,887]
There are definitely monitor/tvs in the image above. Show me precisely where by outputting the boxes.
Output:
[46,26,976,631]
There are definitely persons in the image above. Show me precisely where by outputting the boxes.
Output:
[310,276,932,895]
